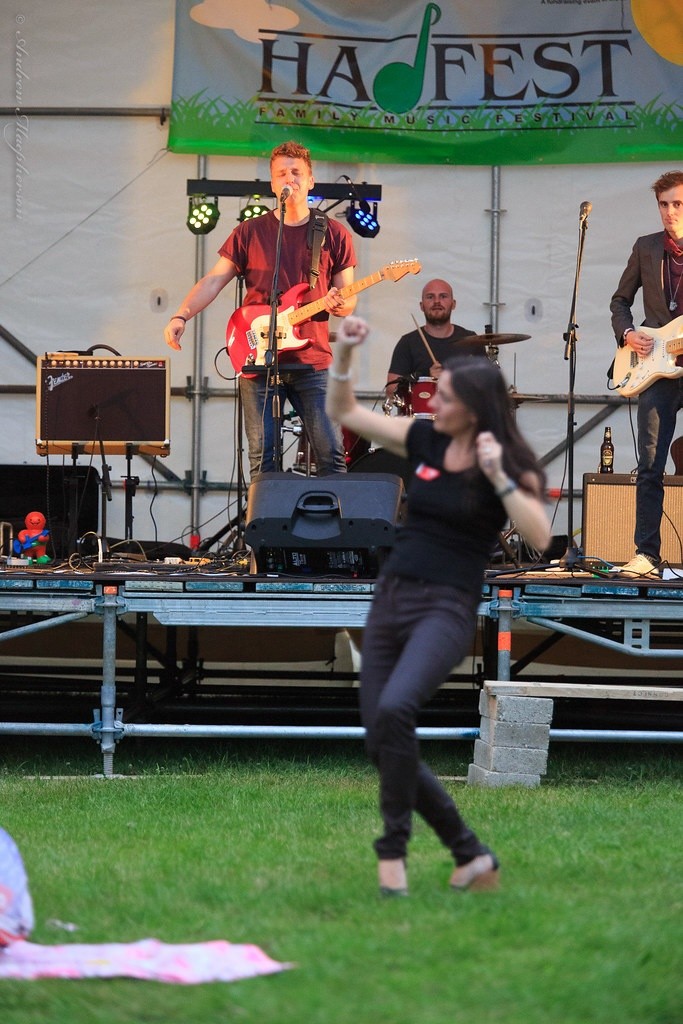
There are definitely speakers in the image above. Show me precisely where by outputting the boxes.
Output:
[582,473,683,572]
[36,354,171,457]
[245,472,406,577]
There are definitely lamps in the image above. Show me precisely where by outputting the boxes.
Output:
[186,179,382,238]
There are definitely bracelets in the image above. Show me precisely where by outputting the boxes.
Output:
[171,316,187,323]
[623,328,634,340]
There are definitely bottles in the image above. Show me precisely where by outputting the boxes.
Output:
[600,426,614,473]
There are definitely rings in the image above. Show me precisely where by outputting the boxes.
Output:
[640,346,644,350]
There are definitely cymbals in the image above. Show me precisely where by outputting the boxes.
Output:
[451,333,531,345]
[509,392,550,403]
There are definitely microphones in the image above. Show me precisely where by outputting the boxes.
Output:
[389,373,419,385]
[279,185,294,202]
[578,201,593,221]
[344,176,371,214]
[88,402,98,417]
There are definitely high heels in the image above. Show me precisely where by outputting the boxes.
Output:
[445,853,500,895]
[375,855,409,902]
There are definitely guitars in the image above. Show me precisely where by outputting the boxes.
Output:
[225,257,422,379]
[613,314,683,398]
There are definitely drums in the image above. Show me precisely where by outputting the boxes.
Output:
[347,447,411,484]
[292,418,372,477]
[394,376,438,421]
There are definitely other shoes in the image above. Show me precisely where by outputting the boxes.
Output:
[610,555,663,582]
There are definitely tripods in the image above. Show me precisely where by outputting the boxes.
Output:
[486,218,617,578]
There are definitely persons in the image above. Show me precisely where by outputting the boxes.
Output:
[164,141,357,486]
[387,279,485,416]
[607,172,683,580]
[327,364,354,381]
[324,315,550,897]
[495,483,518,496]
